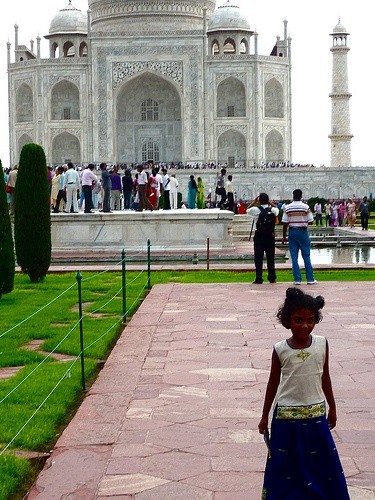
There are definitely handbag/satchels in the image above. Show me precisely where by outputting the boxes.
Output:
[215,187,224,195]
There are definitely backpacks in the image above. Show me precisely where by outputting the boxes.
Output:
[256,205,276,232]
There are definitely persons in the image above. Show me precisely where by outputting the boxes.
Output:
[3,160,186,216]
[196,177,205,209]
[270,199,292,225]
[282,189,317,285]
[223,175,234,211]
[324,198,357,228]
[358,196,370,231]
[187,175,197,209]
[215,168,226,210]
[205,193,211,208]
[213,197,248,214]
[185,161,325,169]
[314,199,323,226]
[257,287,351,500]
[246,193,279,284]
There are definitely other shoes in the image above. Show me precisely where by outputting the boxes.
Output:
[307,282,318,285]
[294,282,301,284]
